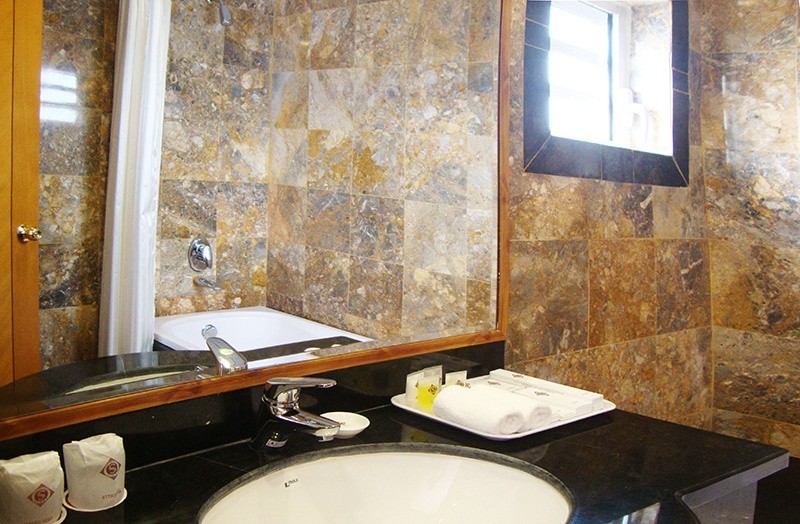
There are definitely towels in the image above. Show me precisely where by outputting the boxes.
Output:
[475,383,551,430]
[434,386,523,437]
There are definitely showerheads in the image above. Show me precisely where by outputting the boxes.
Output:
[207,0,234,26]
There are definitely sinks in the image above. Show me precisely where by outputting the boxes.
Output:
[63,365,218,396]
[192,441,576,523]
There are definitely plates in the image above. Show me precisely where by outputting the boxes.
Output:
[320,412,370,440]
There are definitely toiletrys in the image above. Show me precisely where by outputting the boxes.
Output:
[404,364,468,410]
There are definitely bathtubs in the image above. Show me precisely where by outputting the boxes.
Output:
[153,305,376,351]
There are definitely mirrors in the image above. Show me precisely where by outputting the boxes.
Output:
[0,0,509,444]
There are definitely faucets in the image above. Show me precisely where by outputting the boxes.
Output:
[247,375,343,447]
[195,337,248,378]
[194,276,222,293]
